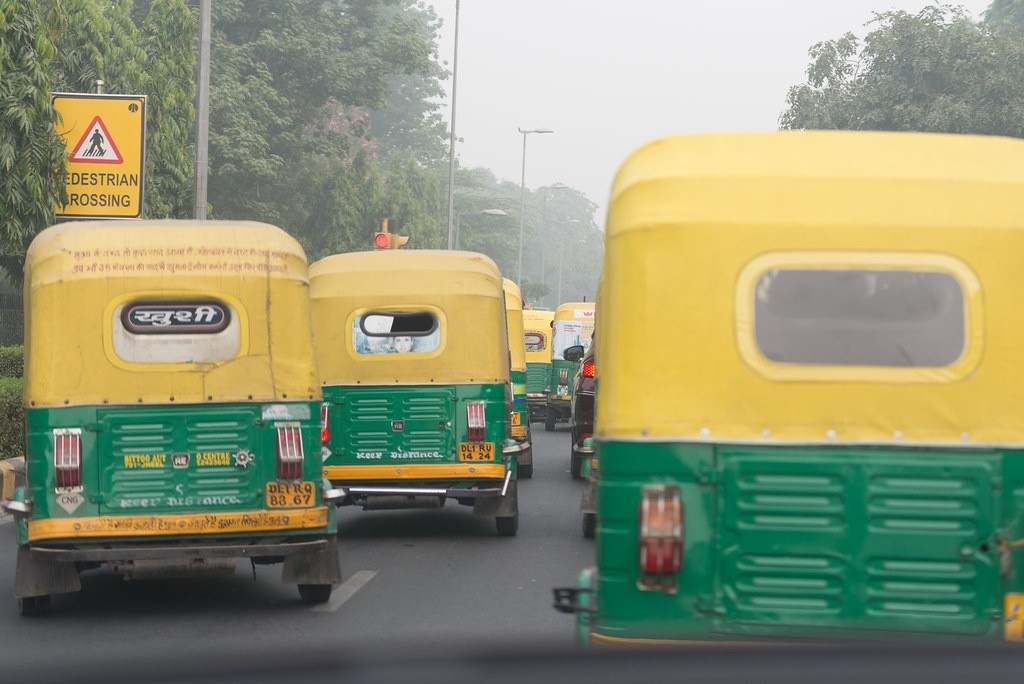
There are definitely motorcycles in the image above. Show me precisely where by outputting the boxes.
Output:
[497,277,597,538]
[548,127,1024,656]
[1,217,341,620]
[302,245,521,538]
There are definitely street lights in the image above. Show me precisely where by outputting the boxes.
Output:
[517,129,555,291]
[456,209,507,252]
[558,215,581,305]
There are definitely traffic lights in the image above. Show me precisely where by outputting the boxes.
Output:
[391,233,409,250]
[373,231,391,250]
